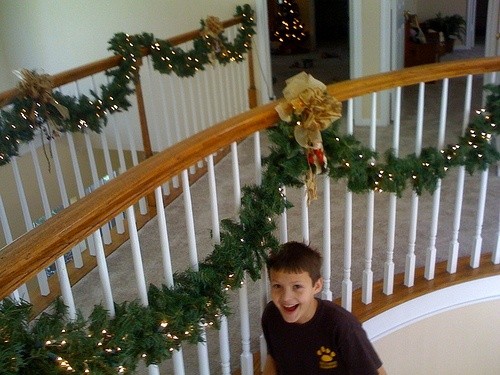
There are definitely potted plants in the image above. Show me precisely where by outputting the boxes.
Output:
[430,12,465,52]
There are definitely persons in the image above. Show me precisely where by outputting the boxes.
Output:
[261,238,389,375]
